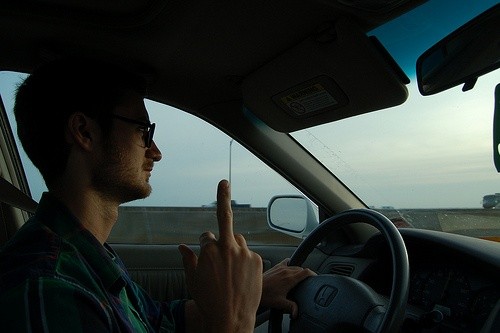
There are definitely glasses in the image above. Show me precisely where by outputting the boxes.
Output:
[91,109,156,147]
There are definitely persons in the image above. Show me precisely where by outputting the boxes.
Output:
[1,51,318,333]
